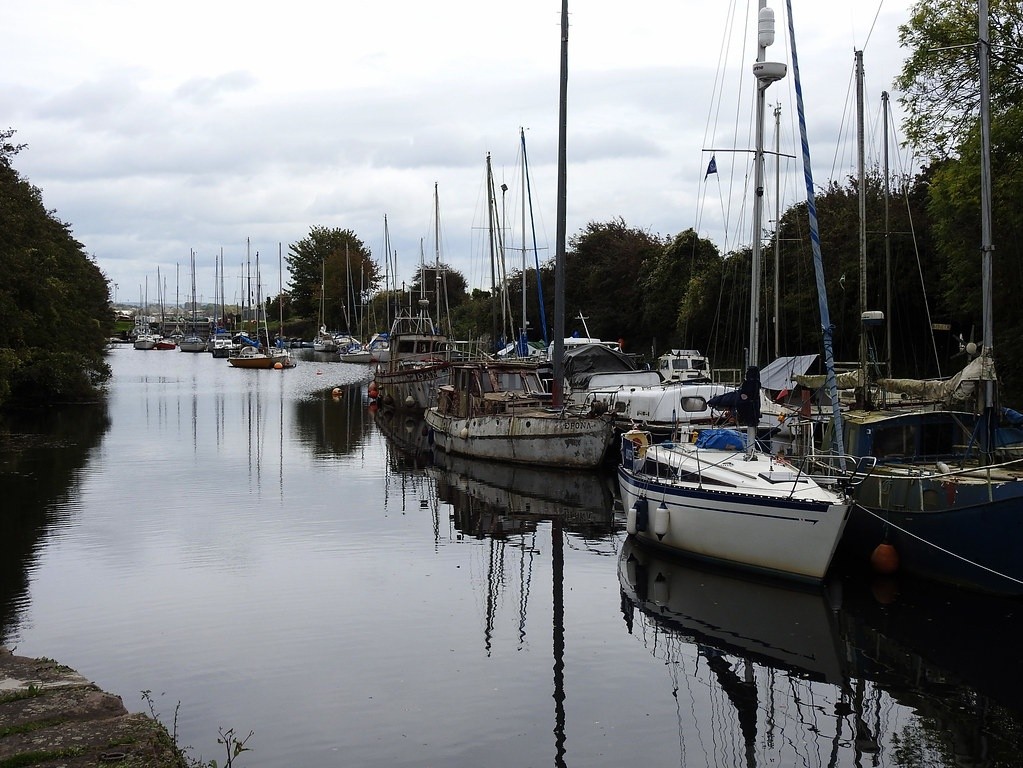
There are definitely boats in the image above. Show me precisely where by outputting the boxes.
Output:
[314,0,1023,582]
[132,237,297,368]
[370,403,855,689]
